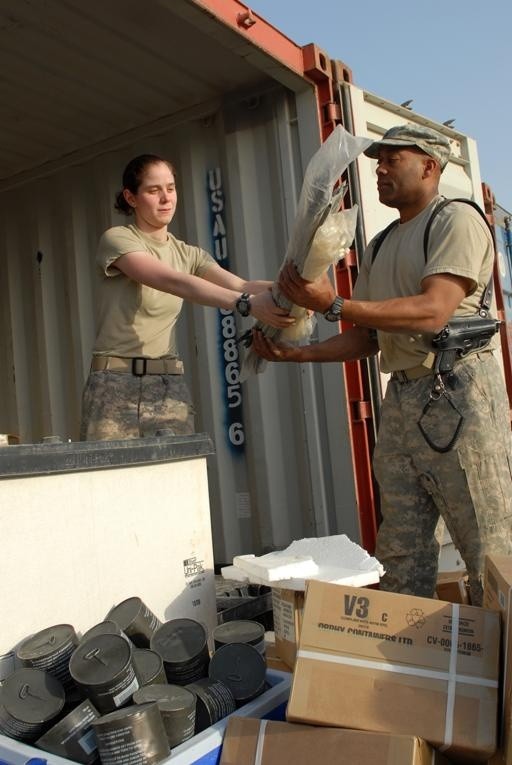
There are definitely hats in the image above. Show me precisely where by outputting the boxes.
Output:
[365,125,451,172]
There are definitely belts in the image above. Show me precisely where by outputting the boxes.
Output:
[393,348,493,385]
[89,355,184,379]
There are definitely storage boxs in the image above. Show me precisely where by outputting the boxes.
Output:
[483,552,511,764]
[0,667,293,764]
[264,641,292,672]
[271,569,386,672]
[284,577,502,765]
[216,583,274,633]
[217,713,435,763]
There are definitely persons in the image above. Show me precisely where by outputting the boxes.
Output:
[246,121,512,608]
[80,154,317,442]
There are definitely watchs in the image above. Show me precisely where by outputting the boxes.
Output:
[324,295,345,323]
[235,291,252,318]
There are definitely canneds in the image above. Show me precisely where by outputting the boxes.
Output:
[1,596,268,764]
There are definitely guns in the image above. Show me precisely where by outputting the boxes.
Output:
[432,316,505,386]
